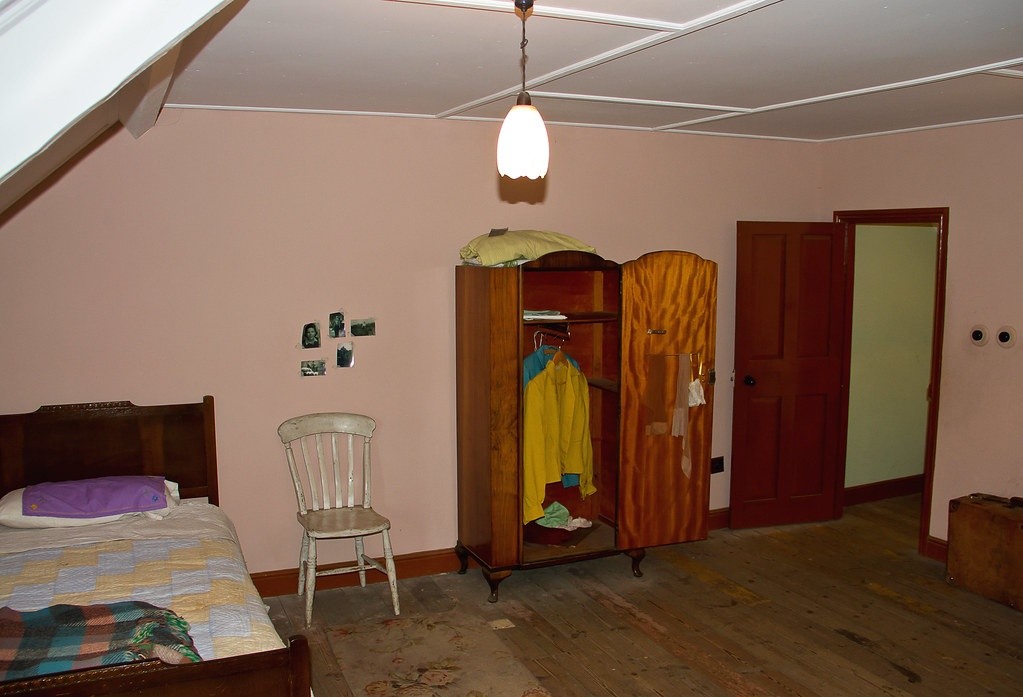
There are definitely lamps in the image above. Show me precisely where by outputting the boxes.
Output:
[495,0,550,181]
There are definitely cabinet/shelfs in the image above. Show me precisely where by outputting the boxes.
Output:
[453,249,718,605]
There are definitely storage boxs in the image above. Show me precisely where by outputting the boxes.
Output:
[944,492,1023,613]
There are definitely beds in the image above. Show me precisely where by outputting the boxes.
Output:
[0,394,315,697]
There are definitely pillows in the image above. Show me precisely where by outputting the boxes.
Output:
[0,476,180,529]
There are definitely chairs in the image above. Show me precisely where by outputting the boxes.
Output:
[277,410,402,630]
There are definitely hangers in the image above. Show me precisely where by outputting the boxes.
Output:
[542,330,558,355]
[551,332,570,368]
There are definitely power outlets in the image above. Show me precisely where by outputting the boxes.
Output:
[710,455,725,475]
